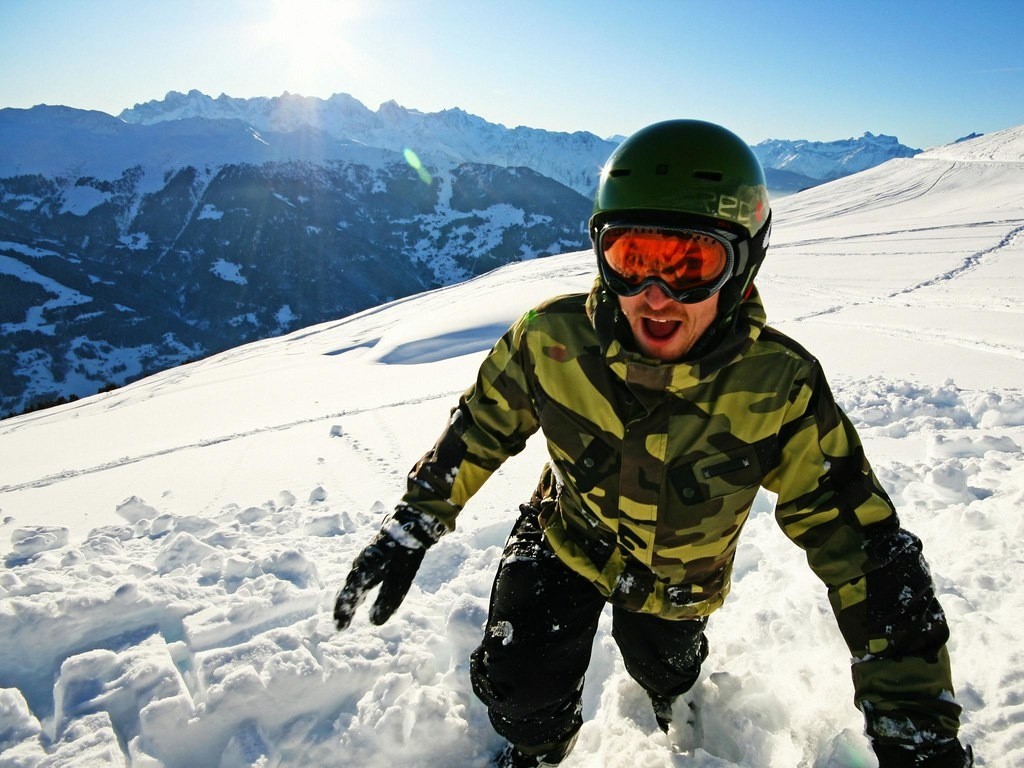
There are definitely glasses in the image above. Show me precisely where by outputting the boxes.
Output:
[594,222,751,304]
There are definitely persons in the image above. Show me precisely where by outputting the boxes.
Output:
[334,119,974,768]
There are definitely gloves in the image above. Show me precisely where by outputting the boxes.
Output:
[332,499,450,632]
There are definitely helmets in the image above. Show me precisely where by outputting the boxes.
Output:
[587,118,773,314]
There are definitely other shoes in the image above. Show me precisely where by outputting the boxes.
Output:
[486,732,579,768]
[650,698,696,735]
